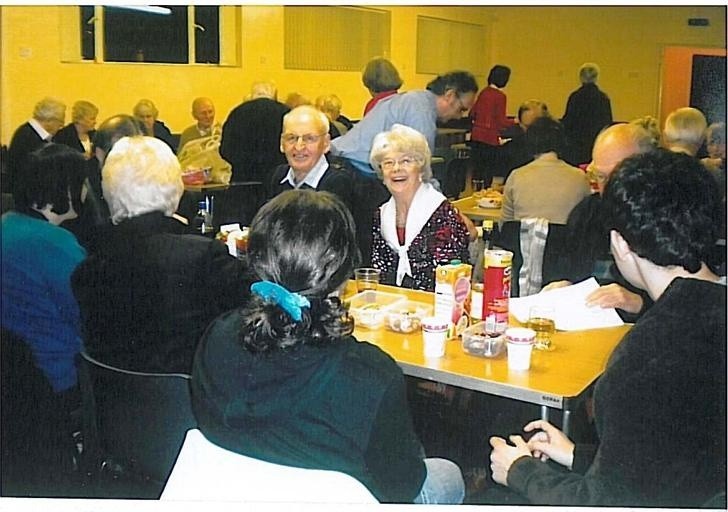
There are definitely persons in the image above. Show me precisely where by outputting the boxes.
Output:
[0,58,726,507]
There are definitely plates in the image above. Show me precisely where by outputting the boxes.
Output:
[477,201,506,209]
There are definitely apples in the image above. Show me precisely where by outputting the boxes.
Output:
[452,303,462,324]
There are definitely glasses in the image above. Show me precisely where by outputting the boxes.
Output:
[456,94,467,113]
[380,154,420,169]
[283,132,327,145]
[590,168,608,184]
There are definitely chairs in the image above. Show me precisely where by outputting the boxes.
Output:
[73,350,193,500]
[442,155,470,201]
[491,220,566,297]
[0,324,90,497]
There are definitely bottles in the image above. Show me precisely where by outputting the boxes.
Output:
[190,201,212,237]
[420,220,555,370]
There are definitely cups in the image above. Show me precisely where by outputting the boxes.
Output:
[353,268,380,294]
